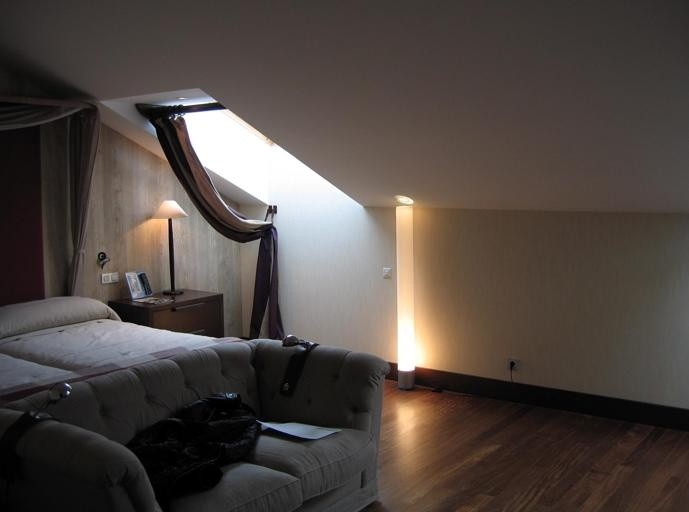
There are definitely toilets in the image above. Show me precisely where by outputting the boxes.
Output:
[125,272,153,300]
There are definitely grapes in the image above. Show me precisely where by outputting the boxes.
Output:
[109,288,225,337]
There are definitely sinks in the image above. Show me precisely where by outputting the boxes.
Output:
[0,336,390,512]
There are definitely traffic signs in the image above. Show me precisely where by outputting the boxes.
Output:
[506,358,520,369]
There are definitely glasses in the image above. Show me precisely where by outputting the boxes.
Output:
[148,199,189,296]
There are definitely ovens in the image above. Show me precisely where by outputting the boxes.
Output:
[0,296,249,403]
